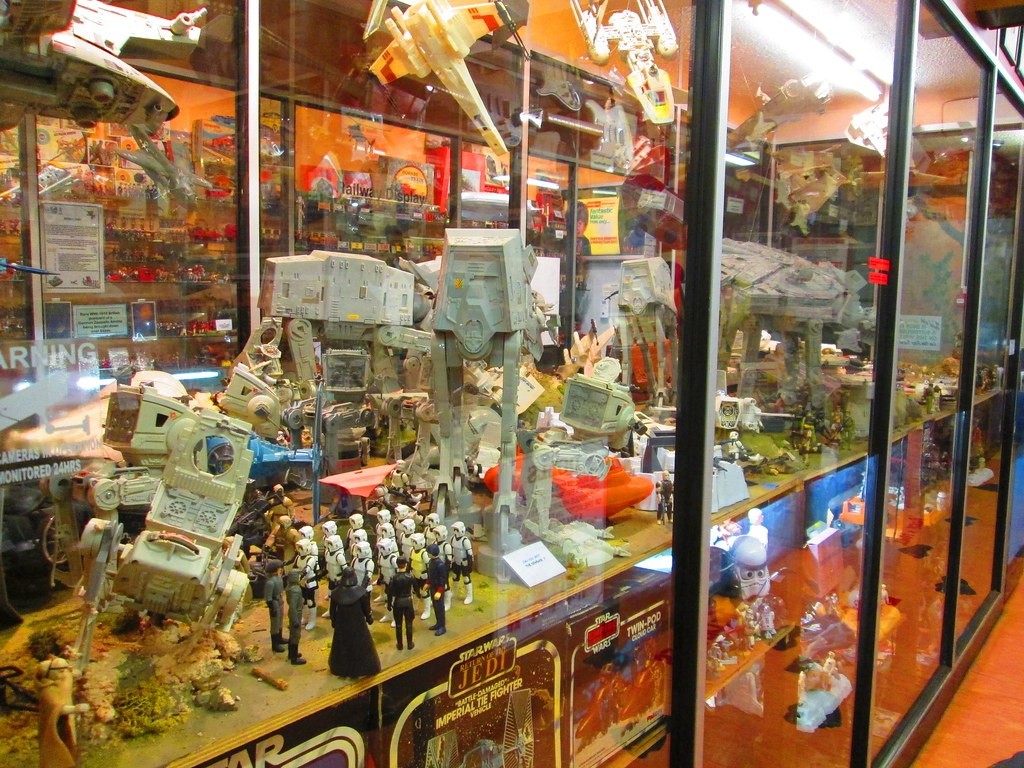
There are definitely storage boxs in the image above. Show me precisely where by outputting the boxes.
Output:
[377,155,434,203]
[495,435,908,695]
[261,244,415,326]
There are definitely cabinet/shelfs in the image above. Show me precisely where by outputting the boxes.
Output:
[298,192,446,253]
[107,238,218,339]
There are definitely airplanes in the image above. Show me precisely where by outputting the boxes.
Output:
[116,125,213,204]
[569,0,679,125]
[368,0,512,157]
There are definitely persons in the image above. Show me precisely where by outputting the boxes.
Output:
[730,535,777,641]
[384,557,421,650]
[326,567,381,678]
[264,559,290,652]
[34,658,91,768]
[576,201,591,258]
[426,544,447,637]
[263,459,475,632]
[286,568,309,664]
[656,470,675,525]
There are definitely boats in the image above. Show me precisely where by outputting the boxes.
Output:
[484,435,655,521]
